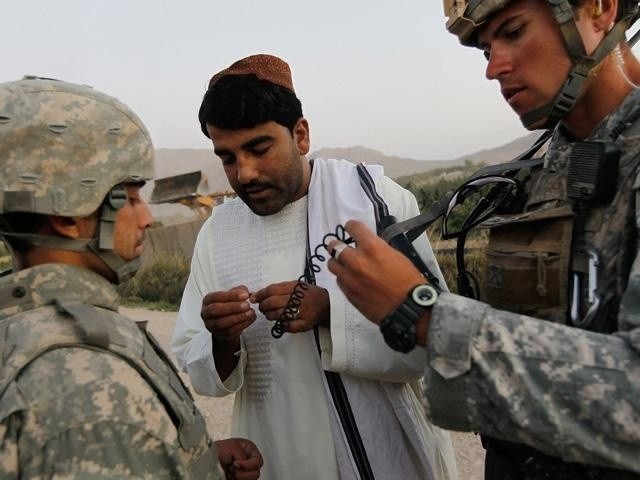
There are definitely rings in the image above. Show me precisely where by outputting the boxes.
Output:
[286,307,299,321]
[330,242,349,262]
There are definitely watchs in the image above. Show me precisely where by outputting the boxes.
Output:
[381,281,444,354]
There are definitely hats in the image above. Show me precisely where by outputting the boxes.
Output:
[208,54,295,93]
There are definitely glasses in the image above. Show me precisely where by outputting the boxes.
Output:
[441,176,519,240]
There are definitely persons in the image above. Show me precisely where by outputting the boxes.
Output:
[168,54,457,478]
[325,0,640,480]
[1,74,265,479]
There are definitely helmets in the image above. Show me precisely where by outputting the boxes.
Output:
[443,0,640,47]
[0,75,155,219]
